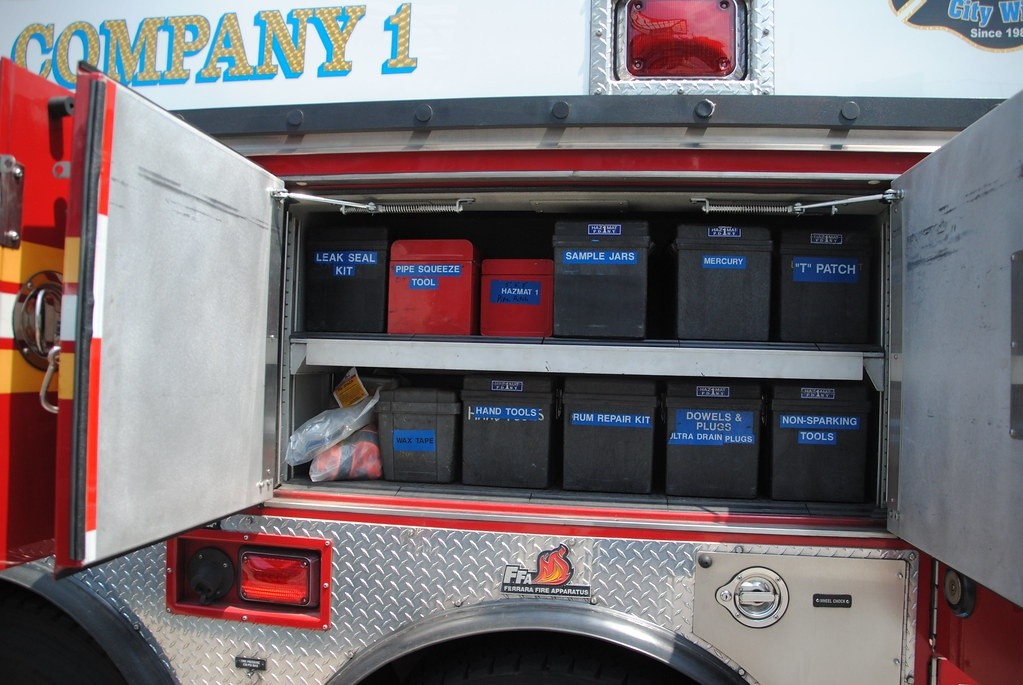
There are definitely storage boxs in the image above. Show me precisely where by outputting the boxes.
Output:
[771,384,873,502]
[387,239,483,336]
[480,258,554,336]
[374,388,463,484]
[361,376,413,395]
[769,227,874,342]
[305,226,400,333]
[562,376,666,493]
[551,220,656,341]
[665,378,765,498]
[675,224,773,342]
[460,374,564,490]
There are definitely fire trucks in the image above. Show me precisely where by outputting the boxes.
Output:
[0,0,1022,685]
